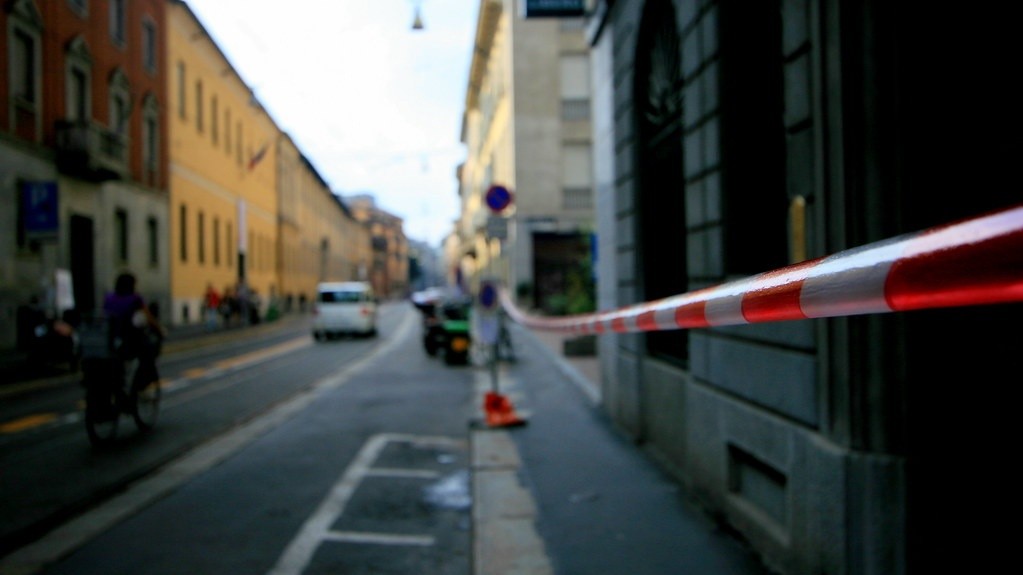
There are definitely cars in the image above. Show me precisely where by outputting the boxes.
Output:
[413,286,444,311]
[311,281,377,342]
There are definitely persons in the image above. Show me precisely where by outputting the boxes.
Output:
[16,289,76,369]
[99,271,164,397]
[200,283,262,331]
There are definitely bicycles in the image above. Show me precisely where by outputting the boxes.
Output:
[85,336,160,447]
